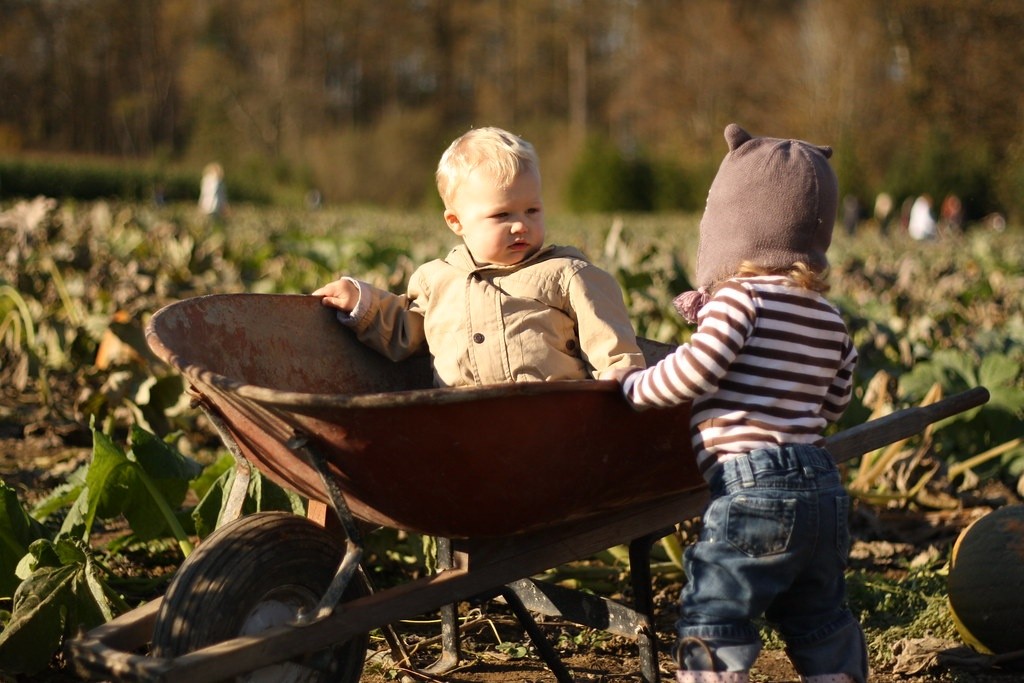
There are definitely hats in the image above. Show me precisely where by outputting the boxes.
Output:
[670,123,838,326]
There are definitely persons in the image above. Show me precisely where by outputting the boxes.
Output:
[309,127,648,389]
[603,135,869,683]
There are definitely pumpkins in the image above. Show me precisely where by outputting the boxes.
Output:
[944,503,1024,683]
[93,309,149,370]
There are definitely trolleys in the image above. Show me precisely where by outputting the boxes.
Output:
[75,291,992,683]
